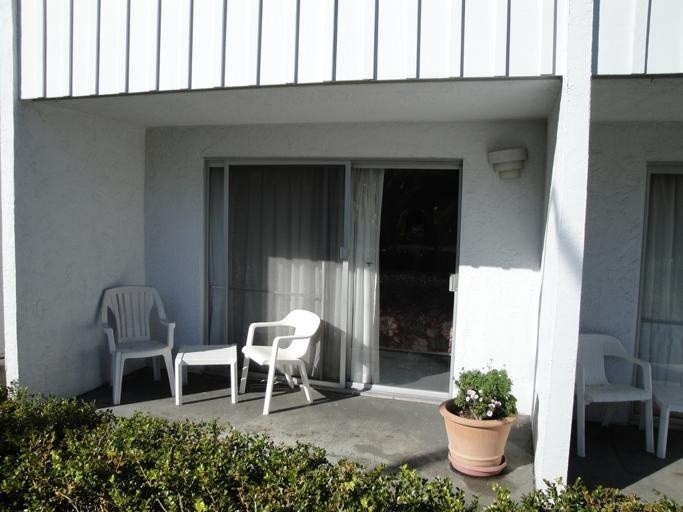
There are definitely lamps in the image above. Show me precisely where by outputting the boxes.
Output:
[488,147,530,181]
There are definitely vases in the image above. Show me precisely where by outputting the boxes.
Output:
[439,398,517,478]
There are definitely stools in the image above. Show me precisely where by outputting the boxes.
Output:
[636,360,681,460]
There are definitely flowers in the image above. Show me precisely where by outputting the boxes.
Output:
[452,358,518,419]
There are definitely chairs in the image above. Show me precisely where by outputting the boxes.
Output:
[574,331,656,458]
[100,285,177,406]
[239,307,324,416]
